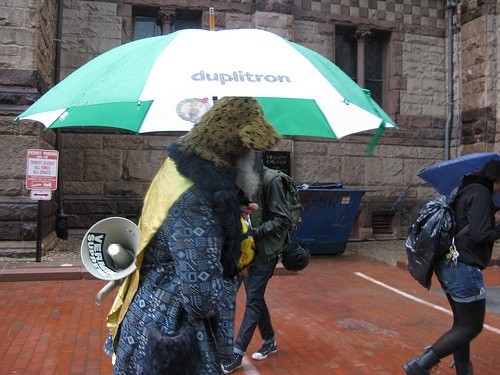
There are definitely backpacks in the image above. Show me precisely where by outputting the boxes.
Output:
[436,182,489,260]
[261,168,305,235]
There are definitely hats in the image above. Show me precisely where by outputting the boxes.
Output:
[178,97,282,175]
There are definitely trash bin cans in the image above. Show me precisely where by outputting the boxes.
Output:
[296,184,375,255]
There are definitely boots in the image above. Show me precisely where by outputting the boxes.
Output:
[402,346,441,375]
[450,360,473,375]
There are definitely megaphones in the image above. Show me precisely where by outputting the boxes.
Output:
[80,216,142,300]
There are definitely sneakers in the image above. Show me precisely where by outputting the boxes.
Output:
[221,352,244,375]
[252,340,277,360]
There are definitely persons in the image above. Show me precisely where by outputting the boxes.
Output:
[220,160,293,374]
[103,96,283,375]
[402,157,500,375]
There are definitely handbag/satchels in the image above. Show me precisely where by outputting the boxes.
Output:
[281,242,311,273]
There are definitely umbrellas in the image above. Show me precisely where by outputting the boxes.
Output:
[13,6,399,140]
